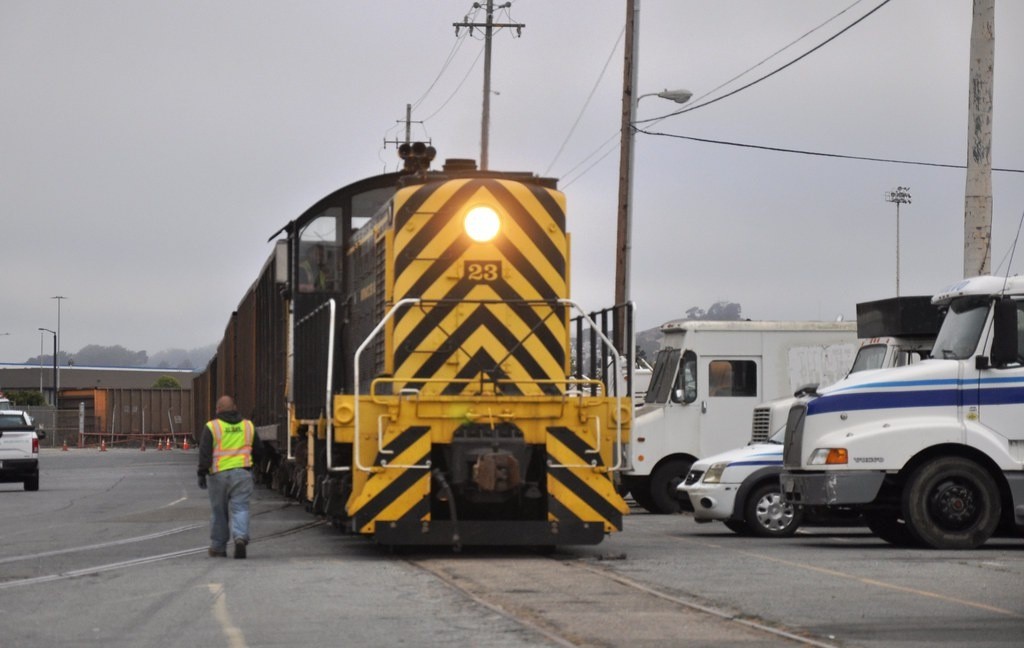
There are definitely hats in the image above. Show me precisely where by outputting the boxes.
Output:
[215,396,237,413]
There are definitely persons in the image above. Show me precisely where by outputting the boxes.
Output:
[197,396,262,559]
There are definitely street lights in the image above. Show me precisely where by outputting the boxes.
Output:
[615,88,693,353]
[51,295,65,390]
[885,186,912,296]
[39,327,57,408]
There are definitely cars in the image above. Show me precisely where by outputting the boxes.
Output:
[674,421,871,539]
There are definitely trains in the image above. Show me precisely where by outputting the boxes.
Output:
[194,142,634,547]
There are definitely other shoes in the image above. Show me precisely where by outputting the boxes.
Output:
[234,541,245,558]
[208,548,227,557]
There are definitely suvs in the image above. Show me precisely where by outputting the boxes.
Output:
[0,409,46,490]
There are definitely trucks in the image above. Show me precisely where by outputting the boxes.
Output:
[749,296,949,443]
[779,275,1024,550]
[568,321,863,513]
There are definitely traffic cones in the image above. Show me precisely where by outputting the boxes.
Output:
[165,437,172,450]
[99,440,107,451]
[62,441,68,451]
[183,437,189,450]
[157,438,163,451]
[140,439,146,451]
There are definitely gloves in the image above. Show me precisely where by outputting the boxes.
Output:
[198,474,208,489]
